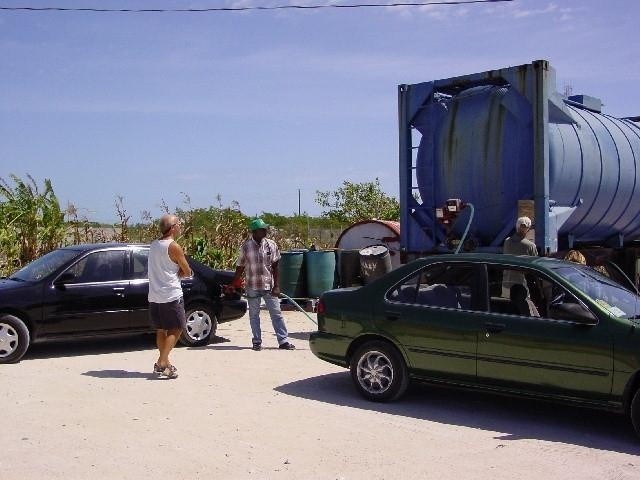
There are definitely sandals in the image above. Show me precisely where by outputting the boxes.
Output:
[154,363,175,370]
[157,368,174,381]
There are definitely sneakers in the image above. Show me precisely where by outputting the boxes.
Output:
[253,343,260,351]
[278,344,295,350]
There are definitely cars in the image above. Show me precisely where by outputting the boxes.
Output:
[310,254,640,434]
[1,245,248,364]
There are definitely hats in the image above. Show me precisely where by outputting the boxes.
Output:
[250,220,269,232]
[516,218,533,226]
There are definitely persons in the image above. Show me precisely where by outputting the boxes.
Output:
[552,251,611,308]
[147,214,192,378]
[227,219,297,351]
[502,216,540,300]
